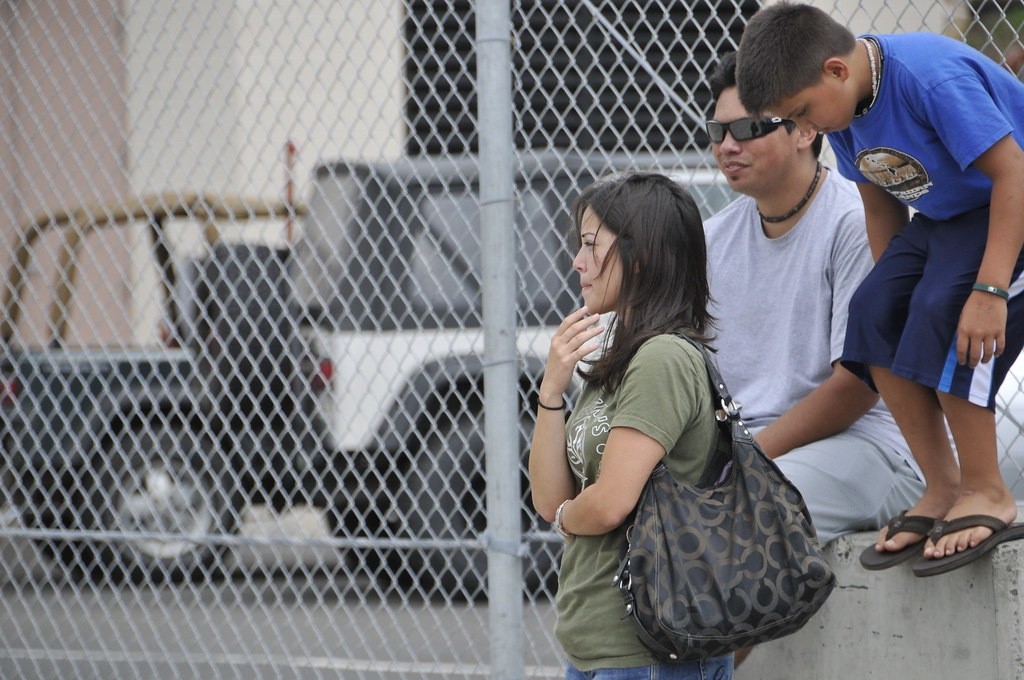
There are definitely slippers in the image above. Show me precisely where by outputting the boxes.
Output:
[859,509,938,571]
[911,514,1024,576]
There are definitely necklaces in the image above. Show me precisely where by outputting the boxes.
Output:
[755,162,822,224]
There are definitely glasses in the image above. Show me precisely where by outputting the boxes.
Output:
[705,116,793,144]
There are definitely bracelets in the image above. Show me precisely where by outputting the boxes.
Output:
[537,396,568,411]
[972,281,1010,301]
[552,498,574,538]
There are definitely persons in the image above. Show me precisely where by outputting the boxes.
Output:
[526,167,839,680]
[692,1,1024,578]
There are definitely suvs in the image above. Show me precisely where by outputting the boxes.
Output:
[292,149,732,599]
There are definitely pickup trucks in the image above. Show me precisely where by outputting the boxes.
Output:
[0,202,309,583]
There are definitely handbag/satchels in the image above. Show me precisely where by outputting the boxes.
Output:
[614,332,836,666]
